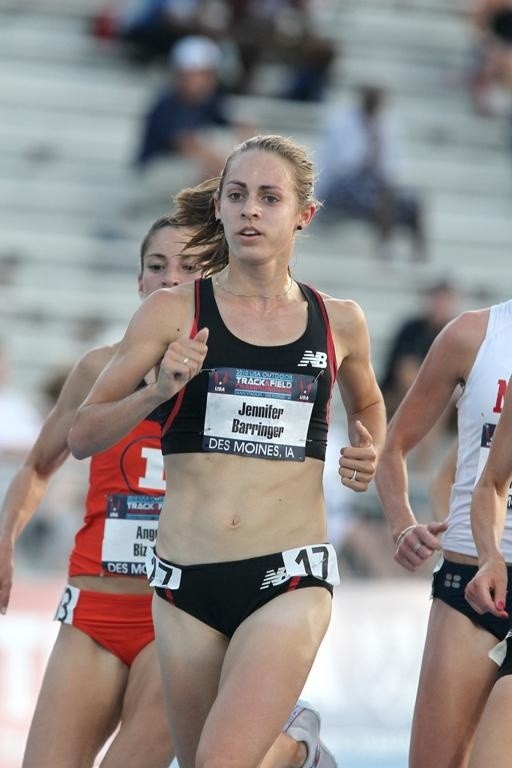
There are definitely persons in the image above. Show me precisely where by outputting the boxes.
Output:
[473,0,511,118]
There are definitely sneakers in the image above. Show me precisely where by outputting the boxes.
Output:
[284,701,337,768]
